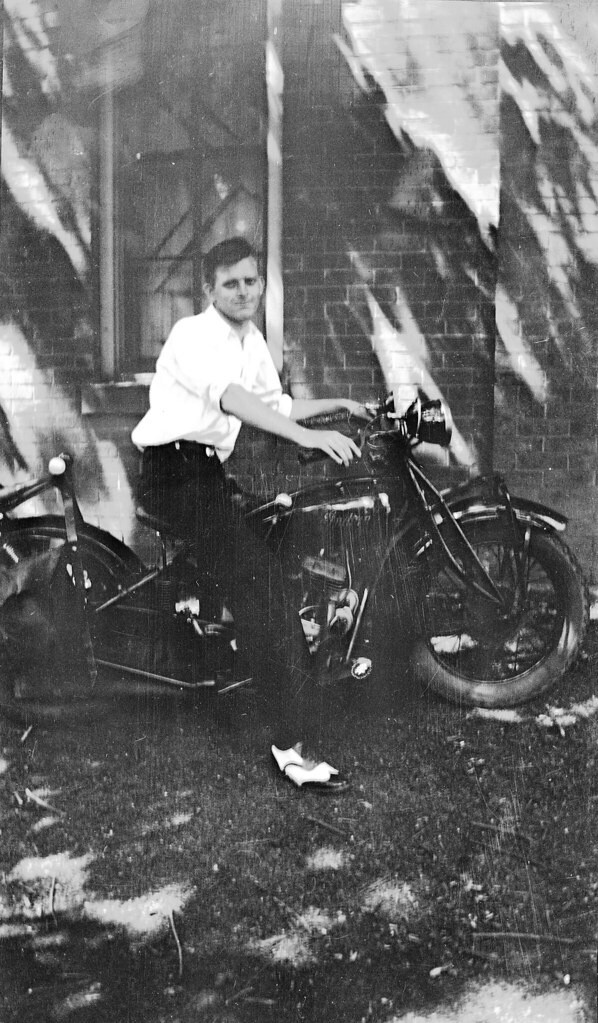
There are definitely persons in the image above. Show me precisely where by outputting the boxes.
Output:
[127,234,376,794]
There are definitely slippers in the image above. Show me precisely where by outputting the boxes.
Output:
[270,742,351,794]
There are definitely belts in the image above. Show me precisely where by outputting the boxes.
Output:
[155,439,217,456]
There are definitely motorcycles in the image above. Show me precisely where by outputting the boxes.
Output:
[0,390,590,727]
